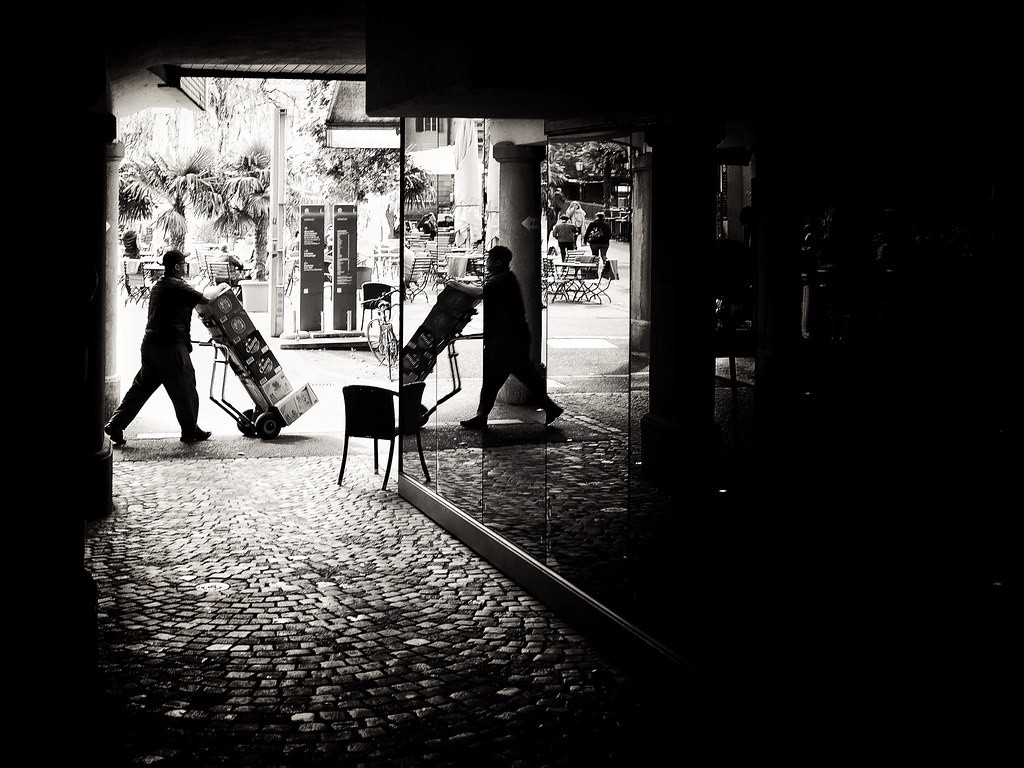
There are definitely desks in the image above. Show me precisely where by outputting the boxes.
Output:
[552,263,596,302]
[445,253,484,276]
[133,254,165,308]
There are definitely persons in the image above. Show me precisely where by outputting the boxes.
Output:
[583,212,613,264]
[446,246,564,429]
[416,213,437,241]
[438,215,454,227]
[553,215,578,262]
[606,193,616,210]
[566,201,586,248]
[541,186,566,242]
[105,250,227,445]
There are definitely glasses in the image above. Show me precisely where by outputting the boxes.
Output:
[178,262,188,267]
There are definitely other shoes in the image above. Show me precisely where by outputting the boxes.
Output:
[545,406,564,426]
[104,423,127,445]
[180,426,212,442]
[460,415,488,428]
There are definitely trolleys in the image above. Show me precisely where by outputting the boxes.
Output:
[199,339,282,442]
[417,328,487,427]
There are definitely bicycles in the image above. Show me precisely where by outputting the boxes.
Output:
[359,290,399,382]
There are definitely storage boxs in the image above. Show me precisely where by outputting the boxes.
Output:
[421,304,465,349]
[247,350,282,386]
[402,342,437,382]
[408,325,446,359]
[436,285,480,319]
[258,371,293,406]
[274,383,320,426]
[193,287,243,329]
[227,330,270,371]
[201,310,256,338]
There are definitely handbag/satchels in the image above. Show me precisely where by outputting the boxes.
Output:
[588,227,603,243]
[581,235,588,247]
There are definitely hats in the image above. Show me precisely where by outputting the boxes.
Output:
[162,250,191,266]
[485,245,513,263]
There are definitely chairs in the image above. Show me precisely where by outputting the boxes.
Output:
[545,259,569,302]
[553,248,586,289]
[116,261,151,305]
[335,385,397,491]
[209,263,234,287]
[406,257,433,303]
[361,282,391,331]
[561,256,596,303]
[403,381,432,484]
[577,260,620,305]
